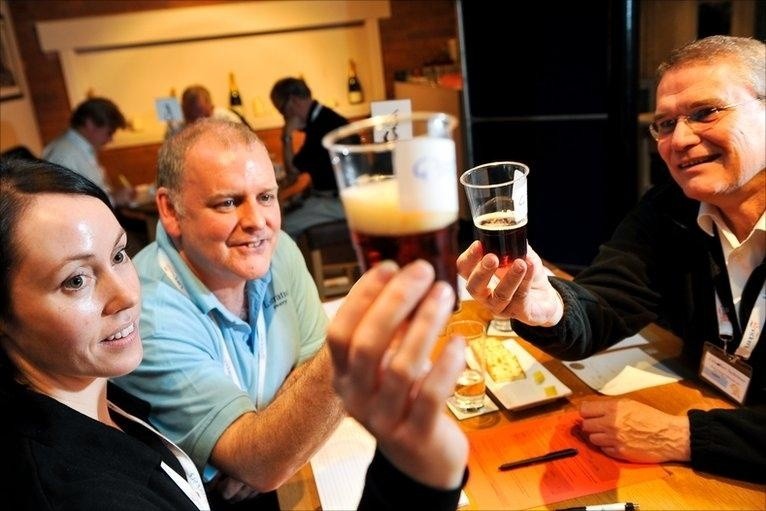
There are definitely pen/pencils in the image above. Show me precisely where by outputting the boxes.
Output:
[499,449,579,471]
[557,503,641,511]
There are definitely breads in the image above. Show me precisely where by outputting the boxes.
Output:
[466,334,526,384]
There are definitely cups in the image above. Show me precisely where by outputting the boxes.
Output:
[447,321,488,413]
[458,161,531,270]
[320,110,463,315]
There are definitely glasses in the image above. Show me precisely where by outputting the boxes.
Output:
[649,98,755,143]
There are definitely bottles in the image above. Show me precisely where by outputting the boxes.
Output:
[346,58,364,105]
[228,73,243,119]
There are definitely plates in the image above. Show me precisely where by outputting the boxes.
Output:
[446,395,500,422]
[462,338,575,412]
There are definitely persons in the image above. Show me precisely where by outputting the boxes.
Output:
[38,97,159,248]
[268,77,372,246]
[0,150,473,511]
[109,114,350,511]
[179,85,245,125]
[454,33,766,486]
